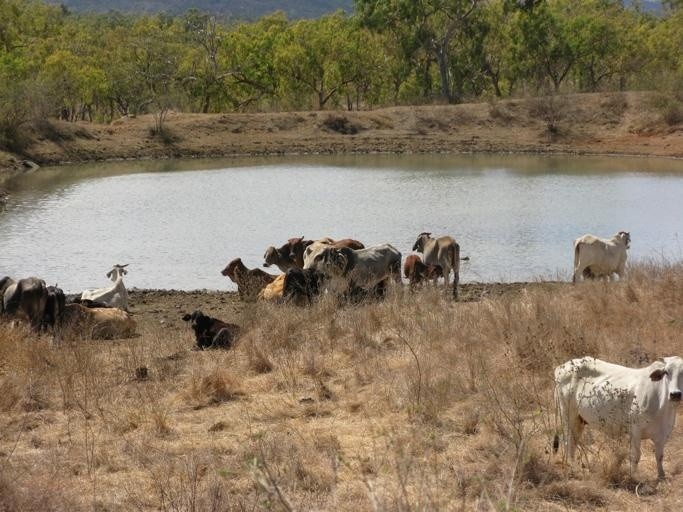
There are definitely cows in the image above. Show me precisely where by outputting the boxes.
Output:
[182,309,241,351]
[572,231,632,285]
[404,254,444,288]
[0,263,137,340]
[413,232,460,302]
[551,355,683,481]
[221,235,403,305]
